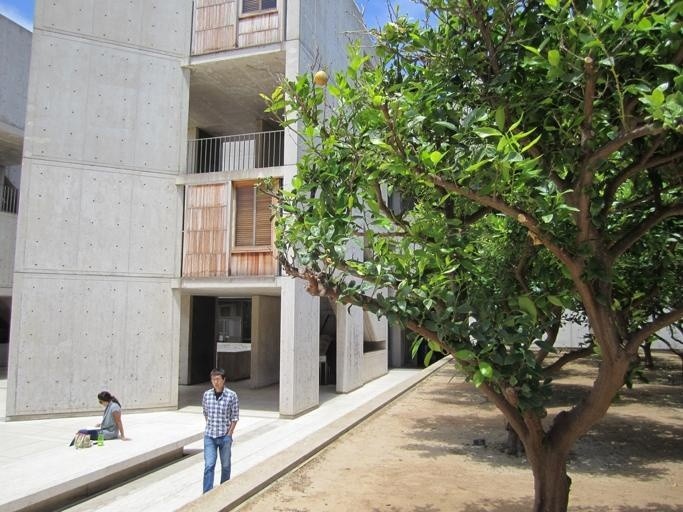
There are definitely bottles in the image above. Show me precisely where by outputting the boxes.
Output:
[97,429,103,446]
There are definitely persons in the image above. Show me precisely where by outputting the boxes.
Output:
[66,391,130,445]
[203,367,240,494]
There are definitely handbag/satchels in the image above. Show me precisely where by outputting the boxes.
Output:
[74,433,91,448]
